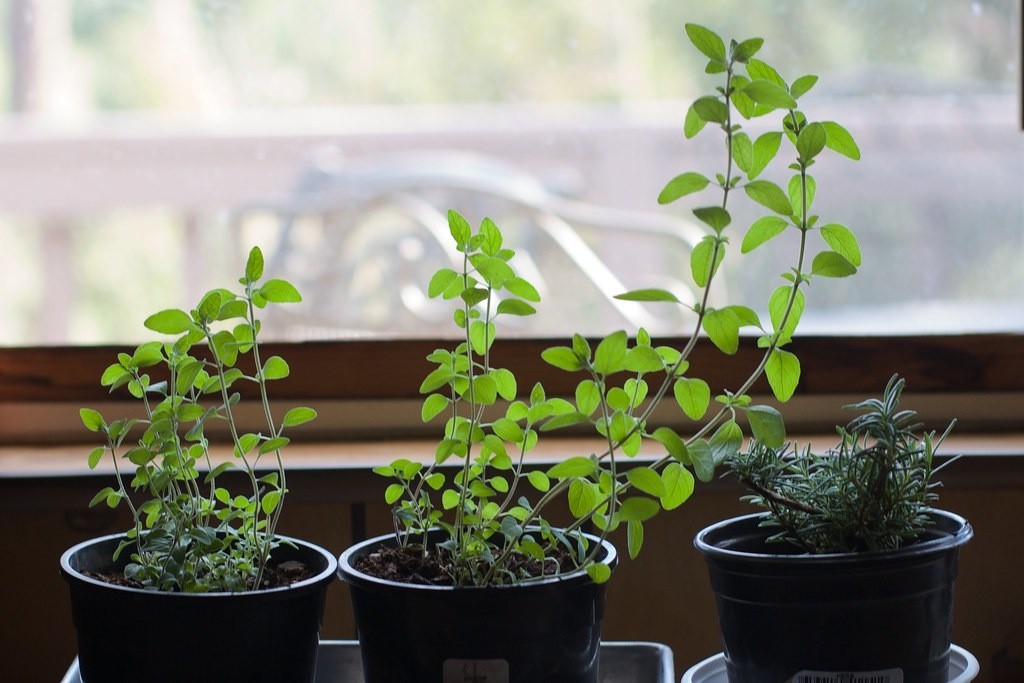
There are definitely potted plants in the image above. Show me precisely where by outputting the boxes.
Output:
[59,244,337,683]
[336,21,861,683]
[694,375,974,683]
